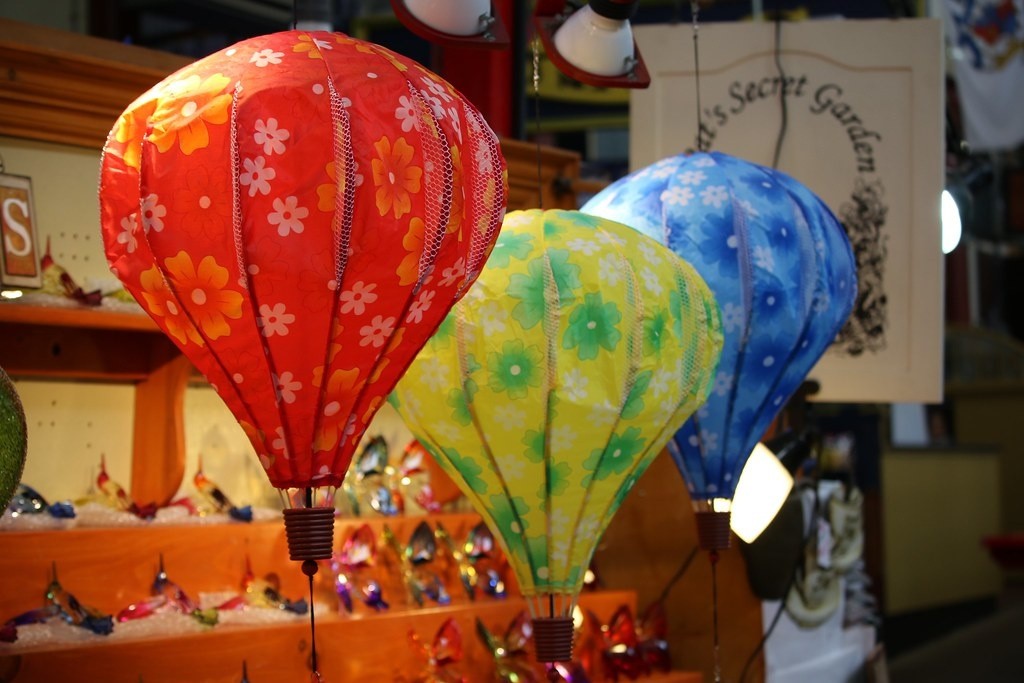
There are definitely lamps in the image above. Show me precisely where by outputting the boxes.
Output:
[704,413,816,546]
